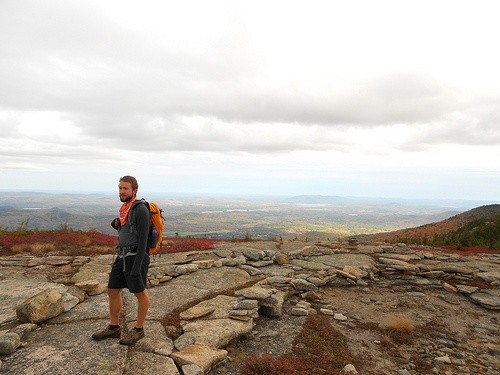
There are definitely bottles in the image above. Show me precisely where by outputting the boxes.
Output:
[152,227,157,243]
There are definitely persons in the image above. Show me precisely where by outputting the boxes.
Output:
[91,176,151,345]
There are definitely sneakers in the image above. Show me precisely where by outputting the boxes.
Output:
[119,328,145,345]
[92,325,120,340]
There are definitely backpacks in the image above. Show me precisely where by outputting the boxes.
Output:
[118,199,166,259]
